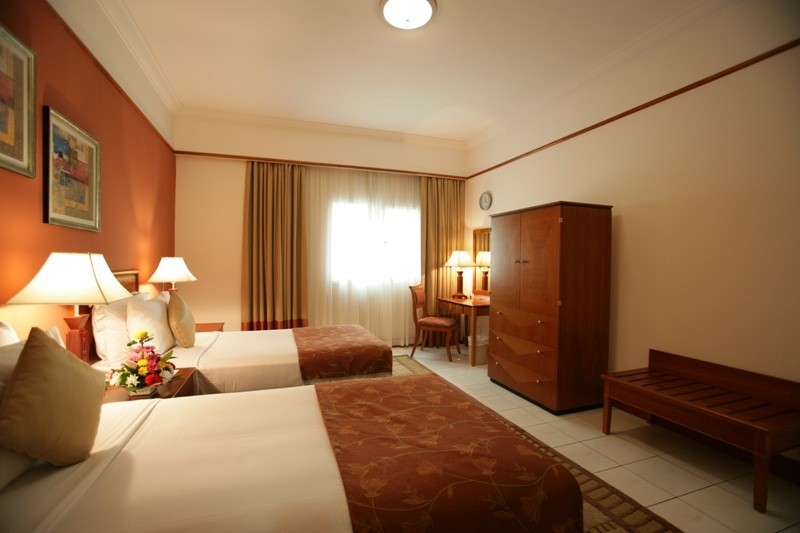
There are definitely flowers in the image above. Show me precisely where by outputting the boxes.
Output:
[105,331,180,392]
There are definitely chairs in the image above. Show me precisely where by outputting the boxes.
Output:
[409,285,461,362]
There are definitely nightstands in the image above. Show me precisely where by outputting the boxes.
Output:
[103,368,196,403]
[196,323,224,332]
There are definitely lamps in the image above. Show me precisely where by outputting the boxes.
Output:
[6,251,132,363]
[444,251,475,299]
[379,0,437,30]
[147,257,198,293]
[476,251,491,291]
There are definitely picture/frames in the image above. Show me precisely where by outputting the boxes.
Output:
[0,23,37,179]
[44,106,100,233]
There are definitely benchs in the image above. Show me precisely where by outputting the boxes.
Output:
[600,368,800,513]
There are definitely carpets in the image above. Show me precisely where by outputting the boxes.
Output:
[393,355,684,533]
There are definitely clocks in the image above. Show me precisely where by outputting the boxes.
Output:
[480,192,491,210]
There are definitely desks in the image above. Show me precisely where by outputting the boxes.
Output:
[436,297,490,367]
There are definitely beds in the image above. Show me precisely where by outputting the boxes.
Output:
[88,268,393,395]
[0,375,582,533]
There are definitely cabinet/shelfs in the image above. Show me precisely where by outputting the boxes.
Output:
[488,204,613,415]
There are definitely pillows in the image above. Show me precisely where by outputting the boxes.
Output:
[92,292,195,364]
[0,323,105,491]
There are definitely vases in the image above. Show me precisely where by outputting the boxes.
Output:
[130,370,178,395]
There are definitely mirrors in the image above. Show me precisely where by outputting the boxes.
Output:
[472,228,491,296]
